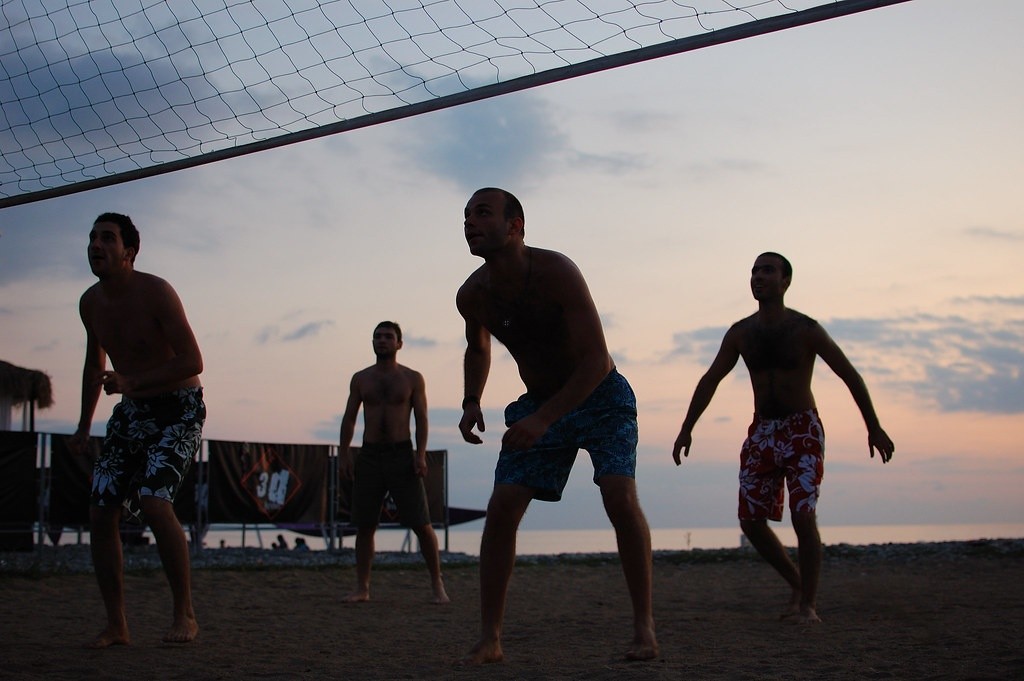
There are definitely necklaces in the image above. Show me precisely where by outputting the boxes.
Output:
[490,248,532,328]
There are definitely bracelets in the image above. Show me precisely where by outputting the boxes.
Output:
[863,410,878,434]
[462,396,481,409]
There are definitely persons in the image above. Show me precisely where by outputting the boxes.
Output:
[455,187,658,671]
[337,322,450,605]
[272,535,288,552]
[294,538,309,551]
[671,252,894,625]
[220,539,225,548]
[65,211,204,648]
